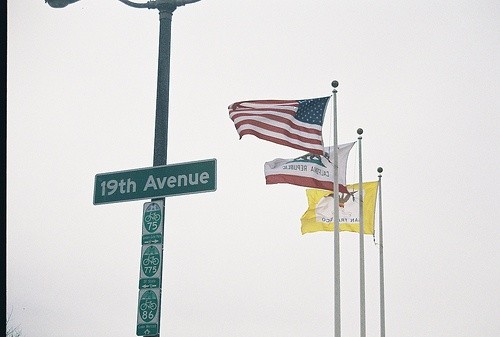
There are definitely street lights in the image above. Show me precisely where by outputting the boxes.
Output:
[46,0,201,337]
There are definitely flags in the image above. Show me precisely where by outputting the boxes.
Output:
[263,140,357,194]
[227,94,331,157]
[300,180,378,235]
